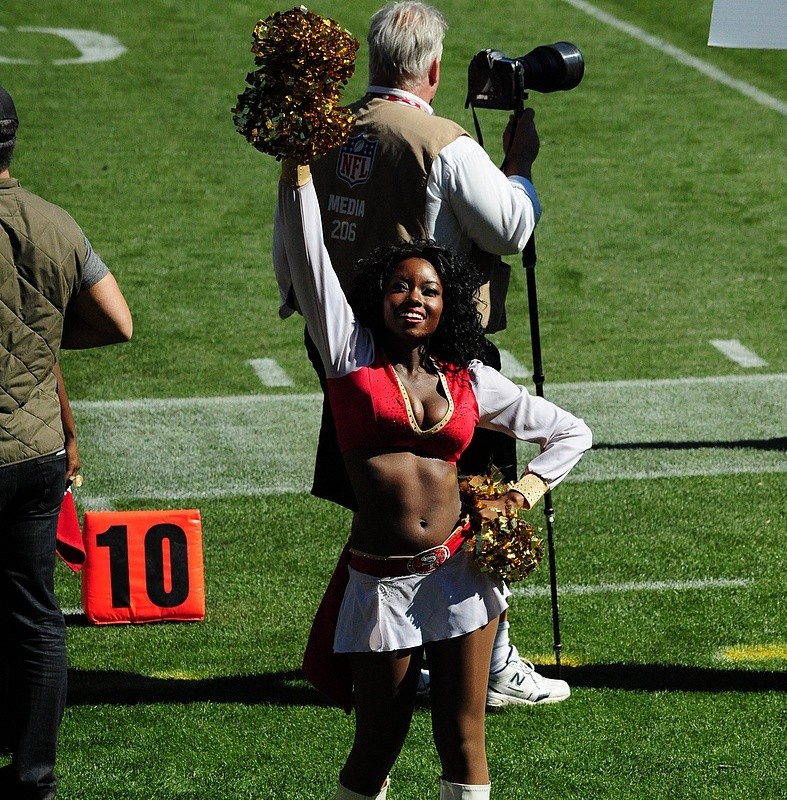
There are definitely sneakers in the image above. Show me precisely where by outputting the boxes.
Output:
[417,666,432,695]
[486,644,571,706]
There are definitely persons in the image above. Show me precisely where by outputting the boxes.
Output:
[274,2,571,710]
[271,156,592,800]
[0,87,134,800]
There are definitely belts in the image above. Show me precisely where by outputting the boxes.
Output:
[349,522,472,578]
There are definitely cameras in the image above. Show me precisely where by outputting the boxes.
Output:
[468,40,585,111]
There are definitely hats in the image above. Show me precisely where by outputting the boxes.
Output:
[0,86,19,120]
